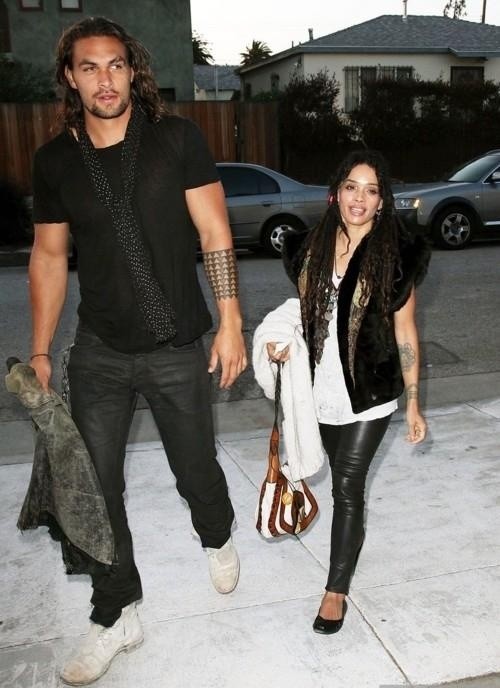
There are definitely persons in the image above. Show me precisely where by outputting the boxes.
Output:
[262,153,435,635]
[29,13,253,687]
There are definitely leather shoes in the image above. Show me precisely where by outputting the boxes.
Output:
[312,600,349,634]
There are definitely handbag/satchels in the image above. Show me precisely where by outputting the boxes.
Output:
[254,471,317,538]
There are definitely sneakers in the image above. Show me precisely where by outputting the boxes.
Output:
[202,535,242,594]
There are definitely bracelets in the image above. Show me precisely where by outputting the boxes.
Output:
[29,353,54,362]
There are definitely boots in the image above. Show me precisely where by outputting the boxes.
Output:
[59,601,145,687]
